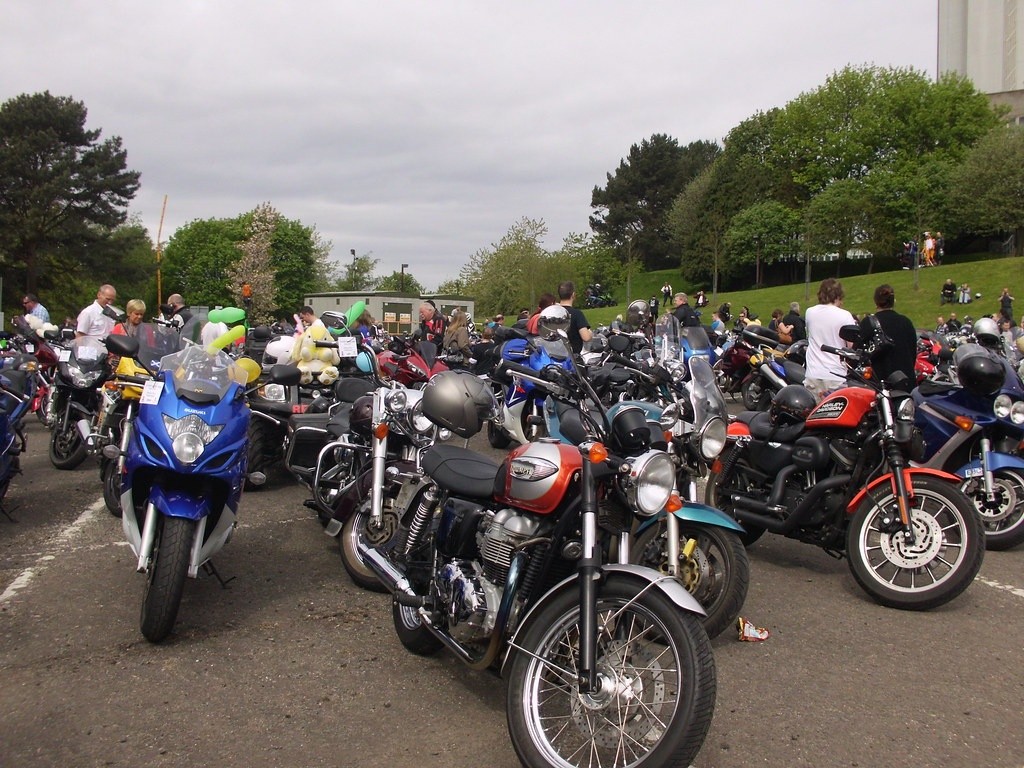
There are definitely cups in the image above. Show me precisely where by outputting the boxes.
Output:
[13,316,18,326]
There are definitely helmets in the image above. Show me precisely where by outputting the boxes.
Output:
[769,384,816,428]
[421,370,494,439]
[958,349,1014,392]
[356,351,378,372]
[973,318,1001,345]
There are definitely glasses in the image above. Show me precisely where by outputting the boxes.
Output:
[23,301,31,304]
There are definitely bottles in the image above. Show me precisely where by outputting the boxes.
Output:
[0,335,19,351]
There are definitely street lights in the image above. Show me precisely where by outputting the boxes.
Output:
[350,249,359,295]
[399,262,408,294]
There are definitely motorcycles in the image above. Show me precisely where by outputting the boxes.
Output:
[0,295,1023,768]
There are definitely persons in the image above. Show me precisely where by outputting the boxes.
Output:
[769,302,806,345]
[512,282,593,354]
[984,312,1024,382]
[737,306,750,331]
[349,309,373,346]
[935,314,962,335]
[23,294,73,330]
[648,282,706,328]
[711,302,732,337]
[273,307,324,334]
[904,231,945,269]
[940,279,971,305]
[420,300,504,382]
[847,284,917,393]
[587,284,602,308]
[998,288,1015,320]
[804,279,855,405]
[76,285,202,370]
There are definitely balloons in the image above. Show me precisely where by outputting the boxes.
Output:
[332,301,366,333]
[206,307,262,384]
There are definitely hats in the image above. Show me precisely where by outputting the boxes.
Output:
[520,308,529,313]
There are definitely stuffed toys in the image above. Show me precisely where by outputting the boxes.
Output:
[29,316,58,338]
[293,326,341,384]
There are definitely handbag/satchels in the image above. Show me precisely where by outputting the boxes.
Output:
[866,312,896,363]
[442,339,464,368]
